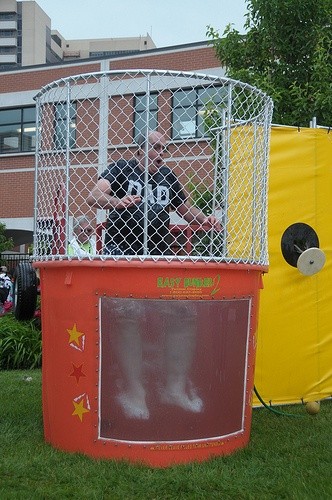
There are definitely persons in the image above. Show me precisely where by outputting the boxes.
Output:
[86,129,224,256]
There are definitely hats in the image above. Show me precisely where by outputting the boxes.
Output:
[0,265,8,271]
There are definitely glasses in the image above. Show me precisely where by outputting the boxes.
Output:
[150,140,170,154]
